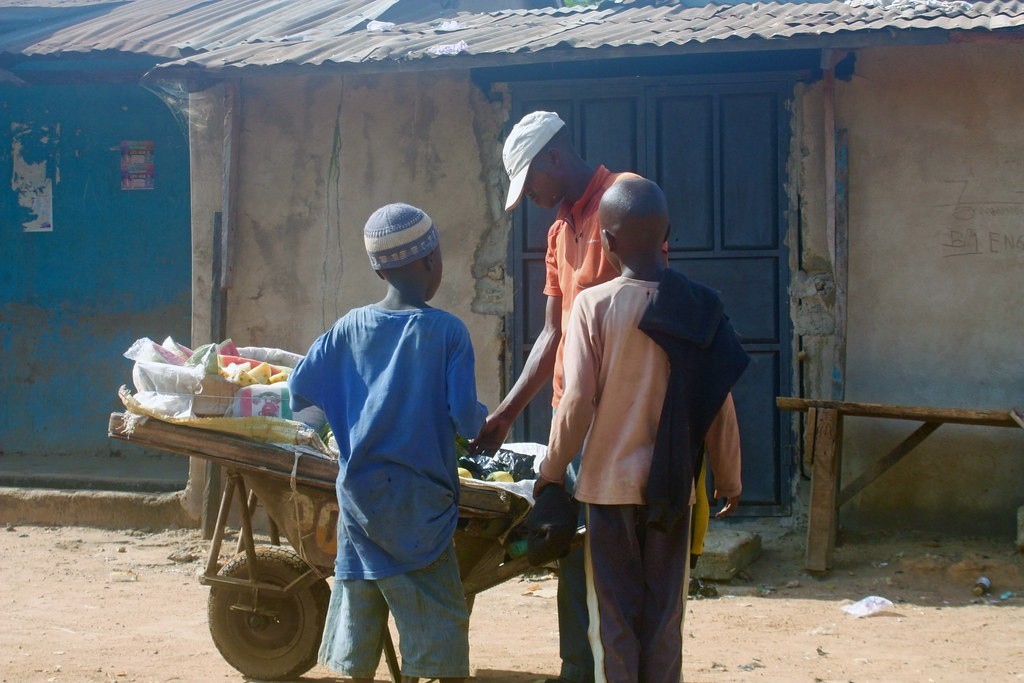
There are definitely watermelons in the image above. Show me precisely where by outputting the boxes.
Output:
[139,335,281,374]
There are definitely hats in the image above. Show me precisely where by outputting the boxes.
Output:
[524,483,580,564]
[501,110,566,211]
[363,202,439,269]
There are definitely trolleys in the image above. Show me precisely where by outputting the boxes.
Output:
[109,408,599,683]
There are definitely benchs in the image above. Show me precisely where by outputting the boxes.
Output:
[772,396,1024,572]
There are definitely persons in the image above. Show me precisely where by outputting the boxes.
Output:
[283,202,495,682]
[530,176,743,683]
[467,107,670,683]
